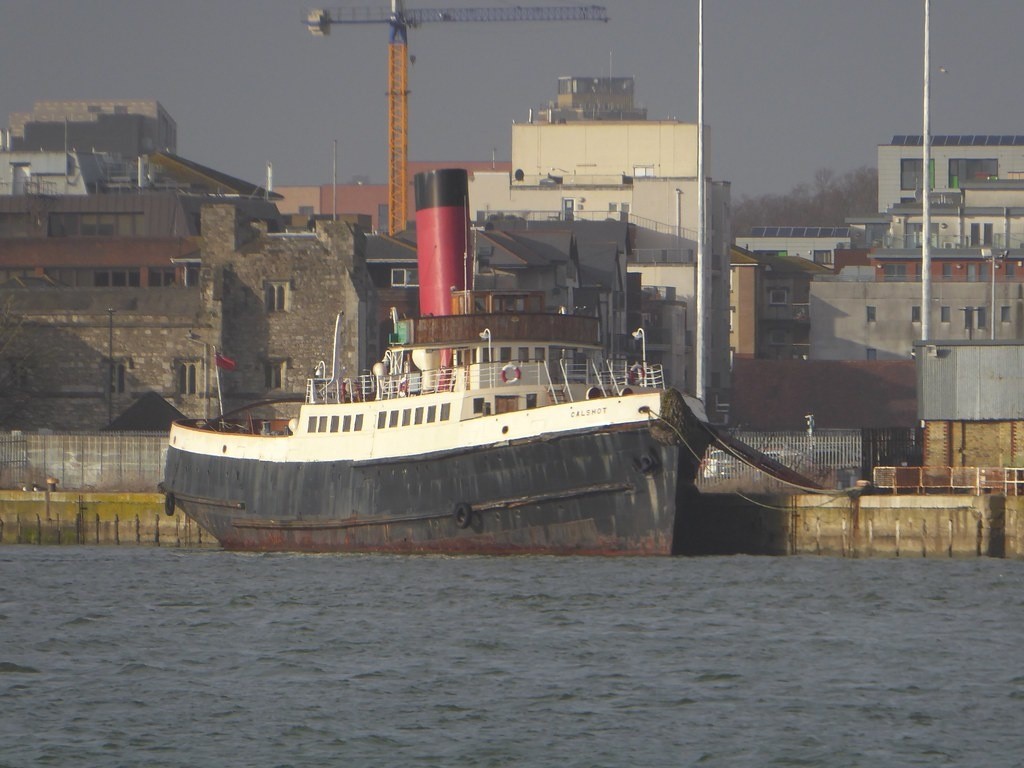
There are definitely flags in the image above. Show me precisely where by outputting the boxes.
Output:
[214,349,236,373]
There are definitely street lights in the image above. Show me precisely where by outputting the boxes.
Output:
[104,305,114,430]
[479,328,494,390]
[381,350,393,398]
[315,361,327,401]
[631,327,648,388]
[979,247,1008,341]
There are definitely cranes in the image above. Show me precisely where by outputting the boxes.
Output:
[302,1,613,235]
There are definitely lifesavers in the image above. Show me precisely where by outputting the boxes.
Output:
[164,492,176,516]
[398,377,408,398]
[500,364,522,386]
[452,502,472,529]
[640,453,654,473]
[630,364,646,386]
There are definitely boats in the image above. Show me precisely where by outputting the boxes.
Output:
[154,196,724,559]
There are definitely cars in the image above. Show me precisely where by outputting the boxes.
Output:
[703,450,746,480]
[751,446,854,483]
